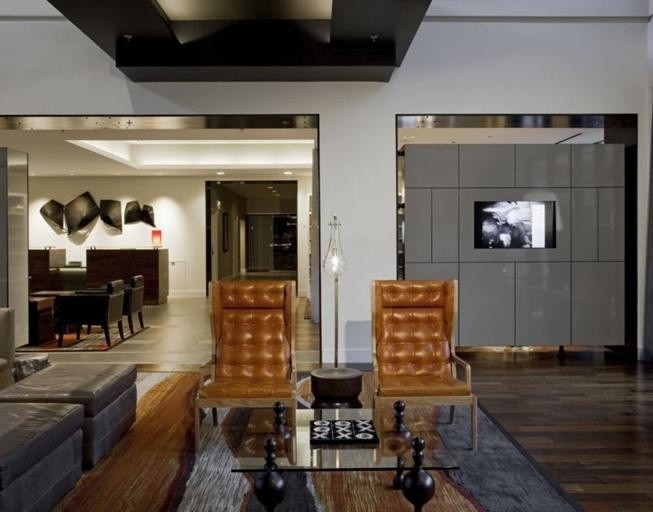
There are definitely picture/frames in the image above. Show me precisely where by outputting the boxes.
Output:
[223,212,229,251]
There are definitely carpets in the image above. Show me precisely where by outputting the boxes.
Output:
[172,370,584,512]
[17,325,148,352]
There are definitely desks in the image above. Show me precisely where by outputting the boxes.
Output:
[30,290,76,296]
[310,368,363,408]
[29,297,55,347]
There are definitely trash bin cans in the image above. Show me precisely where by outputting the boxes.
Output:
[11,355,48,383]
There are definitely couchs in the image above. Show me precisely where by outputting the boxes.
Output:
[0,307,15,389]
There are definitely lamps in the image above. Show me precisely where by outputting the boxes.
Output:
[322,212,346,369]
[152,230,161,246]
[217,200,221,210]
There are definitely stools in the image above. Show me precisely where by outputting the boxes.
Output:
[15,353,50,379]
[1,402,91,512]
[1,362,136,473]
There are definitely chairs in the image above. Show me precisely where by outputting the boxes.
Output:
[194,280,299,457]
[371,279,477,453]
[54,279,124,347]
[87,275,144,335]
